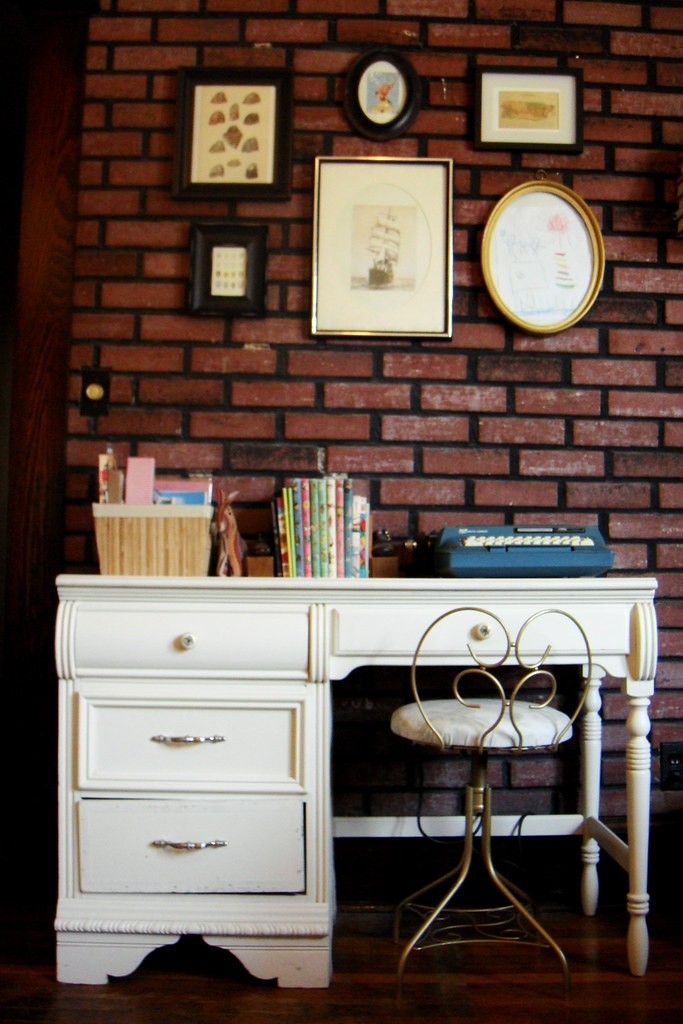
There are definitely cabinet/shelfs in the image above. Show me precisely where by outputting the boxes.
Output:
[53,571,661,989]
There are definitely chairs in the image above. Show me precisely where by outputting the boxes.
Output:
[390,605,593,999]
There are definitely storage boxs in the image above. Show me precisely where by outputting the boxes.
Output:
[91,501,215,576]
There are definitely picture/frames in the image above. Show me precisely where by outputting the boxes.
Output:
[467,63,584,155]
[185,220,269,318]
[171,64,292,202]
[308,154,456,339]
[341,47,423,143]
[478,170,606,336]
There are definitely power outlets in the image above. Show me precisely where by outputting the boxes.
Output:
[659,741,683,791]
